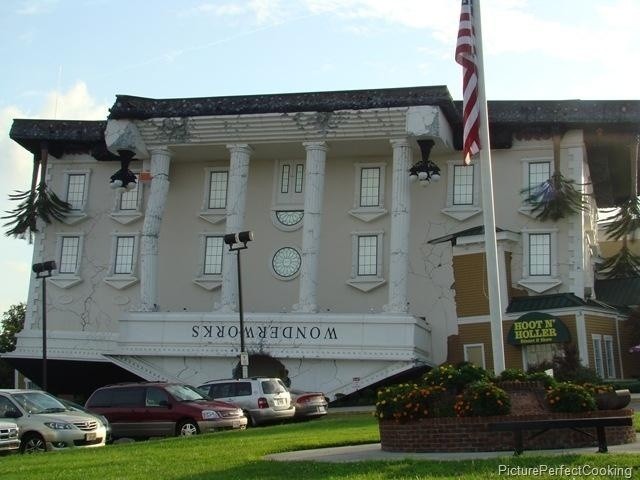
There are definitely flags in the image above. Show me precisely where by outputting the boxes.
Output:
[454,0,482,169]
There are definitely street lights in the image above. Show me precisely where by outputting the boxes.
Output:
[32,260,57,392]
[224,230,254,378]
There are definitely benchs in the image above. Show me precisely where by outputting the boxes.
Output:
[487,416,633,455]
